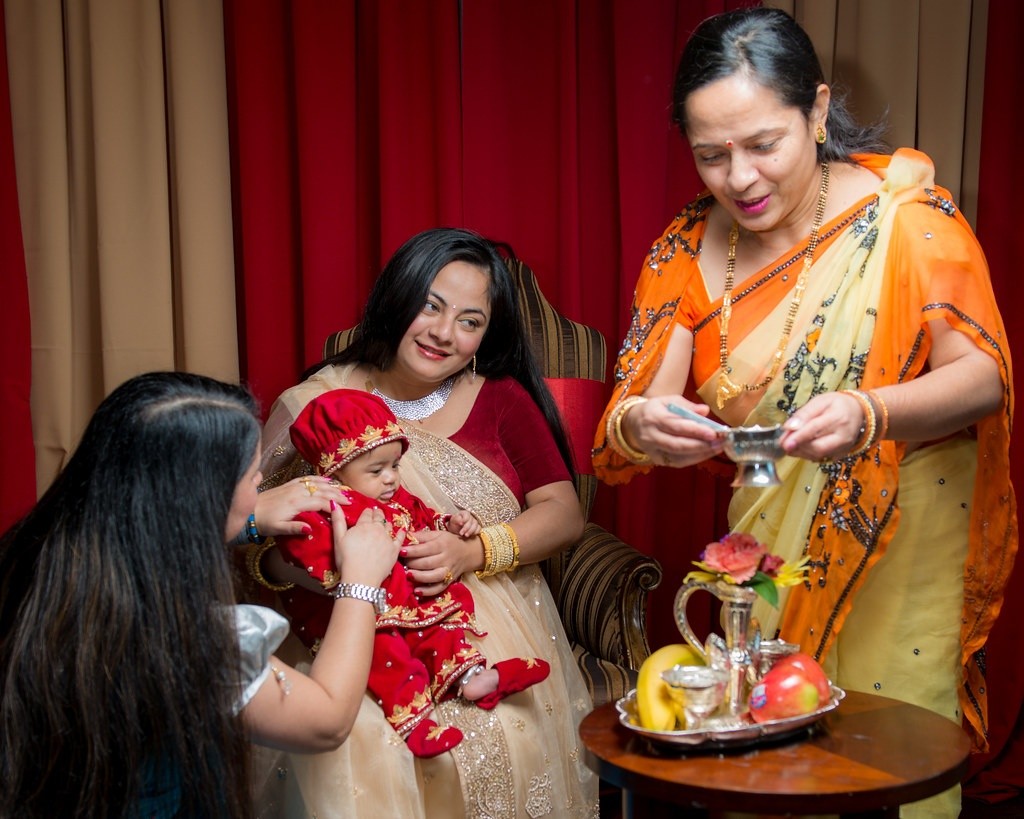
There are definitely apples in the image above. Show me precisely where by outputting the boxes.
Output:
[747,652,831,723]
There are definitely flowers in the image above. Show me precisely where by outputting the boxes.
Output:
[682,529,813,594]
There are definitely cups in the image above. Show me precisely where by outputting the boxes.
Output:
[660,664,731,732]
[716,427,788,489]
[747,641,801,684]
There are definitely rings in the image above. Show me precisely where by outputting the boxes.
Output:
[299,477,311,489]
[308,486,319,497]
[386,530,397,540]
[444,570,455,586]
[664,451,670,467]
[373,519,387,527]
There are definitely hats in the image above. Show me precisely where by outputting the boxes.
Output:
[289,389,410,477]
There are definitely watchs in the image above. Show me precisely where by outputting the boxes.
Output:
[336,583,390,615]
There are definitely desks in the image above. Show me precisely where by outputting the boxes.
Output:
[577,690,976,819]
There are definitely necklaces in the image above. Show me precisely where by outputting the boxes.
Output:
[365,374,456,421]
[715,161,829,412]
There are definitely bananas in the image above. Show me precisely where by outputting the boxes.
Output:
[636,643,706,732]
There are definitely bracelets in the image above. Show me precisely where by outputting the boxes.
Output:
[244,536,296,591]
[232,510,262,546]
[605,397,656,468]
[475,522,520,582]
[830,387,889,462]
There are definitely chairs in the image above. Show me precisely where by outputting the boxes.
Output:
[234,254,662,696]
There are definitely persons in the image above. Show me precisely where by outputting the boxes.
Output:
[0,371,407,819]
[224,227,585,819]
[590,6,1006,819]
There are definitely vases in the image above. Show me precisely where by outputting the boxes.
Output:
[709,577,765,727]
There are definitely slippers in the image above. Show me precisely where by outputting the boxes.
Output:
[476,657,550,711]
[407,718,464,759]
